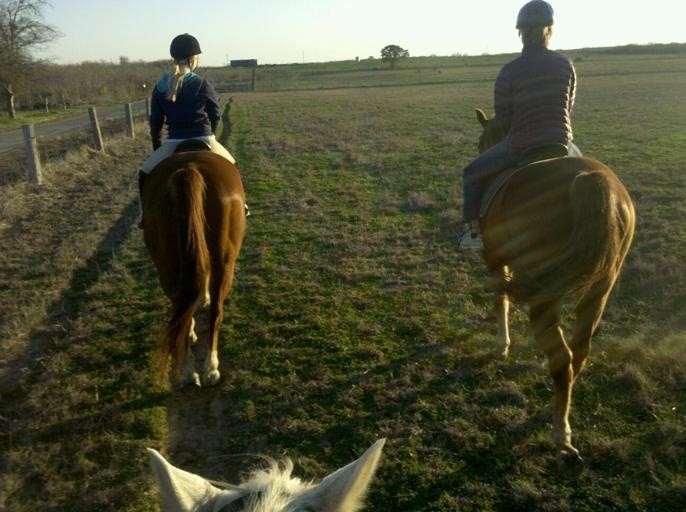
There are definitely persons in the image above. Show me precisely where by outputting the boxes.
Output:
[458,0,577,251]
[137,34,250,229]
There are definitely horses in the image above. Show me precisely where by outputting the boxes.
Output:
[472,106,636,480]
[144,436,388,512]
[140,148,247,390]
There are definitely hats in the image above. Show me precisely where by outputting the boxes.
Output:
[169,33,202,59]
[514,0,556,30]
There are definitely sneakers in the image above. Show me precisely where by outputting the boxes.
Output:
[136,212,143,230]
[455,222,484,251]
[244,203,250,217]
